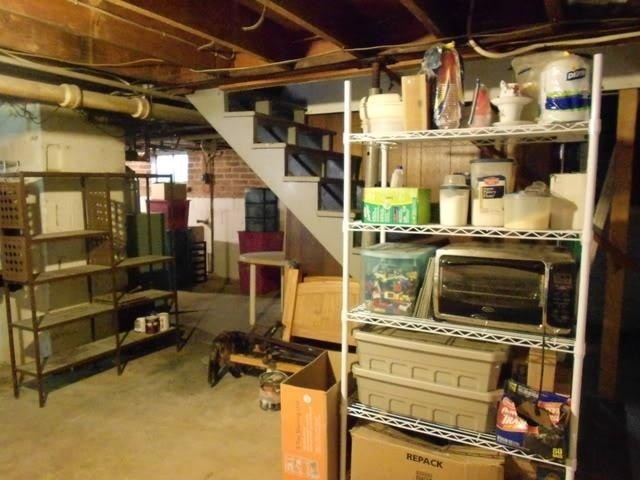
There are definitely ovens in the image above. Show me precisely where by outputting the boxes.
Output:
[433,245,576,341]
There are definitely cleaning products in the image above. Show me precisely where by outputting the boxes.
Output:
[389,165,403,187]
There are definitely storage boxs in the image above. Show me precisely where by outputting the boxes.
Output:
[145,183,191,230]
[280,324,511,479]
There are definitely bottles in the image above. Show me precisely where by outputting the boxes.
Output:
[439,157,588,231]
[391,164,405,191]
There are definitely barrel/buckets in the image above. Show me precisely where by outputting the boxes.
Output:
[245,186,278,231]
[259,372,286,412]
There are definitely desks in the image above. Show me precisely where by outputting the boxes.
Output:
[239,250,283,326]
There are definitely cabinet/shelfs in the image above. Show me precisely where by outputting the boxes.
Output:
[0,171,181,407]
[338,52,604,478]
